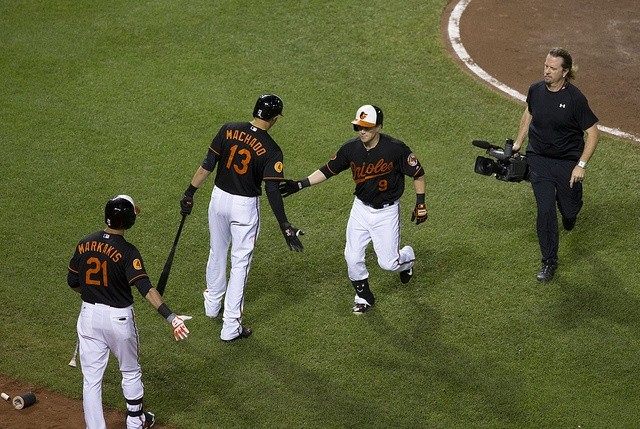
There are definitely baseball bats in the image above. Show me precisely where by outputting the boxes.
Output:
[156,215,186,296]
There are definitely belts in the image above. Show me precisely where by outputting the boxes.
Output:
[373,201,394,209]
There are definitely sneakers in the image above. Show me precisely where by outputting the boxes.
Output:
[352,304,369,315]
[562,217,576,231]
[142,411,156,429]
[233,326,252,339]
[400,267,413,284]
[537,262,557,281]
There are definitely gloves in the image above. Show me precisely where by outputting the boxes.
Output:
[180,190,193,217]
[411,202,428,225]
[280,222,305,252]
[166,312,193,341]
[278,179,303,198]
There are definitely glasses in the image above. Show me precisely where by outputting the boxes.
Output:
[354,124,370,131]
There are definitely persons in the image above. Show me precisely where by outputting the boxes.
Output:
[511,47,599,282]
[280,105,428,315]
[180,92,305,341]
[68,195,193,429]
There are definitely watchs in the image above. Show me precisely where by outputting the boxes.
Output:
[576,160,587,169]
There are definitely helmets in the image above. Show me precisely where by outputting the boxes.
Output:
[105,194,136,230]
[252,93,284,122]
[351,105,383,127]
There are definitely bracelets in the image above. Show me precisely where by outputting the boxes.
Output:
[157,303,171,318]
